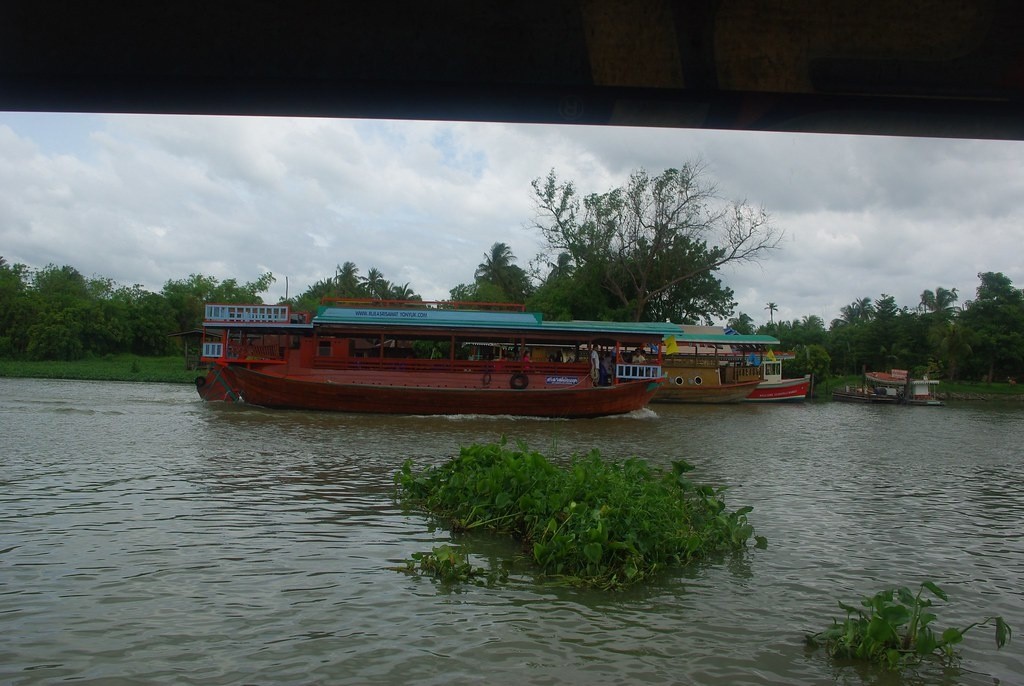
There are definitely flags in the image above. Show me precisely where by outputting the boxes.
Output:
[766,348,776,362]
[648,342,658,353]
[664,335,680,355]
[748,352,760,367]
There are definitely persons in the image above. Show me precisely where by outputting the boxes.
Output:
[524,351,533,370]
[589,344,659,386]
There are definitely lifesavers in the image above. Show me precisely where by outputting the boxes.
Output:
[694,376,703,385]
[510,373,529,389]
[195,376,206,387]
[675,376,684,385]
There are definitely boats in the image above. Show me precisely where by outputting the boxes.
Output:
[906,373,943,406]
[861,368,907,402]
[671,341,812,401]
[451,333,770,401]
[194,303,684,417]
